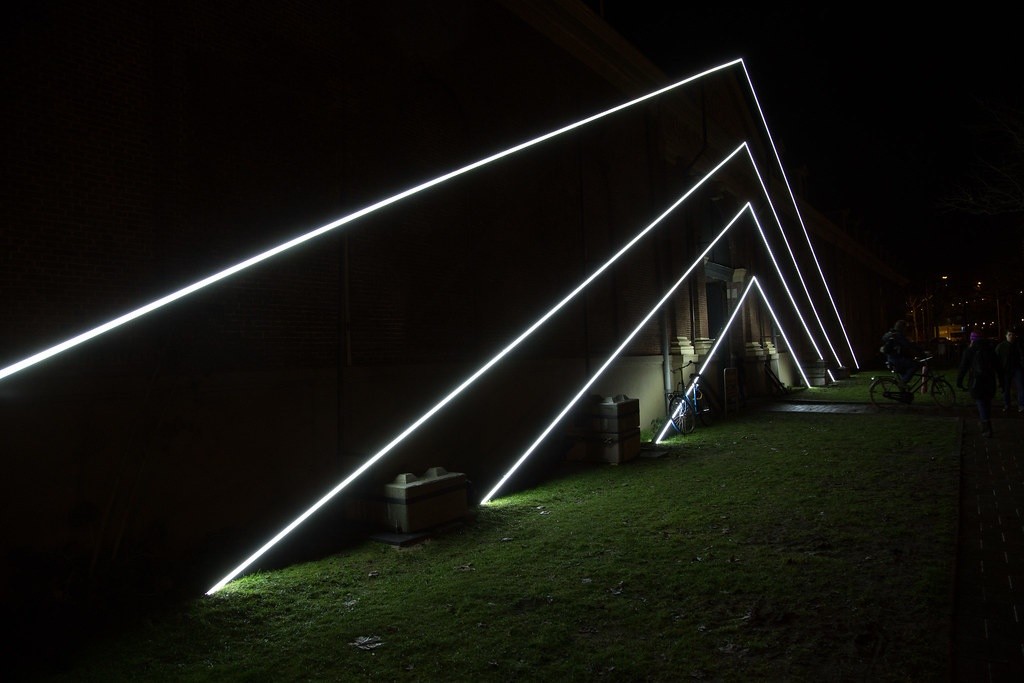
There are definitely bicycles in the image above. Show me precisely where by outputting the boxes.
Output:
[868,349,958,414]
[668,359,717,434]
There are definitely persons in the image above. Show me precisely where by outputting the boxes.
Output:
[994,328,1024,416]
[883,320,922,389]
[956,330,1005,438]
